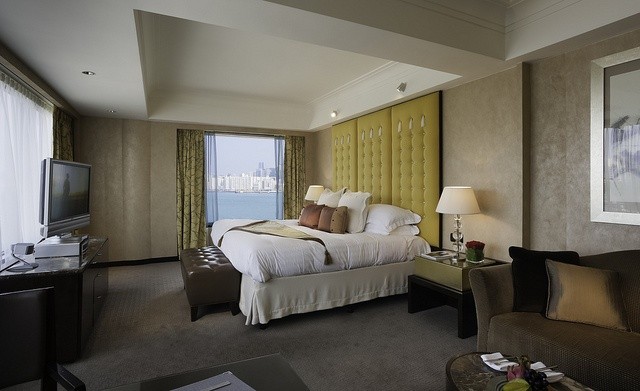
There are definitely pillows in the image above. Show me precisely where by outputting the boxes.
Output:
[318,207,335,231]
[330,206,348,233]
[390,225,421,235]
[318,187,348,208]
[509,246,580,313]
[360,203,422,235]
[337,191,371,233]
[546,257,631,332]
[298,205,325,229]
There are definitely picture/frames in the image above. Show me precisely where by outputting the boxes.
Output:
[590,45,640,227]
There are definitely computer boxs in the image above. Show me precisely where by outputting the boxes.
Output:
[34,235,90,259]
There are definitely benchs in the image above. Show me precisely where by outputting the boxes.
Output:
[180,246,242,322]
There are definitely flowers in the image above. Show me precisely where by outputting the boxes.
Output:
[465,240,485,249]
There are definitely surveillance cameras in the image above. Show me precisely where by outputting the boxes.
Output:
[8,244,40,271]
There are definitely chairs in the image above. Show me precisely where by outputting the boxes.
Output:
[0,286,85,391]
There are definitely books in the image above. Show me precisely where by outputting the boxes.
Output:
[421,251,458,261]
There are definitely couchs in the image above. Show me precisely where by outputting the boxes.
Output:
[211,187,433,329]
[469,249,640,391]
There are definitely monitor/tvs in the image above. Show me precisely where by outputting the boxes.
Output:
[39,158,92,239]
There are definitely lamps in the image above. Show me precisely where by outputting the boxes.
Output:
[305,185,324,205]
[435,186,481,258]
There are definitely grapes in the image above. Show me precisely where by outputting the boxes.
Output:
[525,369,549,391]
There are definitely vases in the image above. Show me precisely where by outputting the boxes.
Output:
[466,249,484,261]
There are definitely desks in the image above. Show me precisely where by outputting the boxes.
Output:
[407,274,479,339]
[1,237,110,364]
[100,353,309,391]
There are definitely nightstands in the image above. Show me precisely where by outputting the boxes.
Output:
[415,249,494,292]
